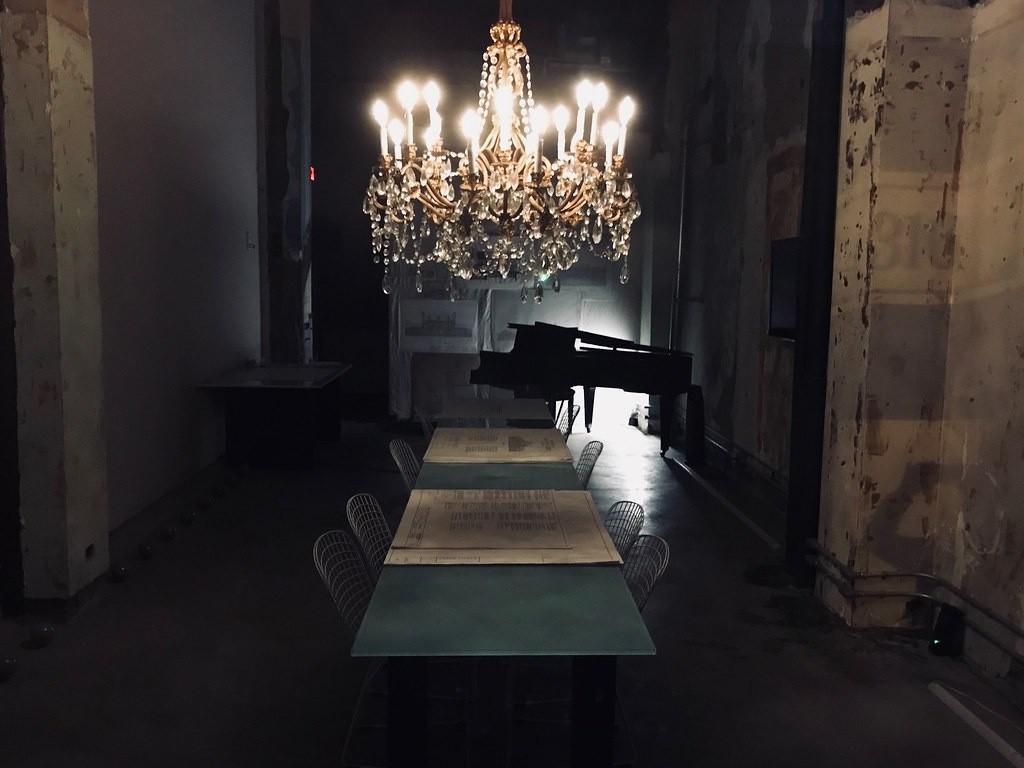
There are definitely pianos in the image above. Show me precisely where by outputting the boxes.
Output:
[469,319,696,458]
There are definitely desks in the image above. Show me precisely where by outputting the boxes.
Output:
[350,419,657,768]
[195,361,353,468]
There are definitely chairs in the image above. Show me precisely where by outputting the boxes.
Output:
[558,405,580,444]
[313,529,473,768]
[346,493,394,581]
[415,404,434,444]
[603,501,645,566]
[502,534,670,768]
[389,439,421,494]
[575,441,603,491]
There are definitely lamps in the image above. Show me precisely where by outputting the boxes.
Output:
[363,0,640,304]
